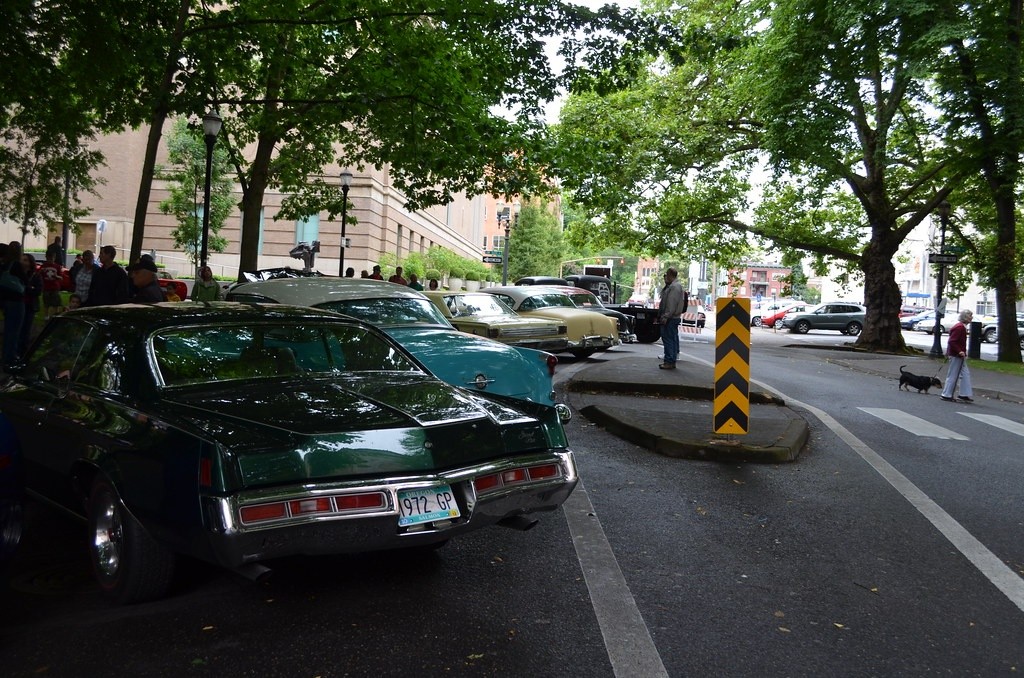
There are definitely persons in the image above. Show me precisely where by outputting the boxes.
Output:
[164,282,181,302]
[89,245,128,307]
[63,294,81,312]
[191,266,221,301]
[939,309,975,403]
[345,265,441,292]
[125,255,168,302]
[657,268,688,369]
[39,249,63,326]
[0,241,41,362]
[70,250,101,306]
[48,236,65,268]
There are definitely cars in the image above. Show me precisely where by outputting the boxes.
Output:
[0,296,578,603]
[415,289,567,353]
[514,274,662,345]
[900,305,1024,350]
[678,305,706,328]
[166,276,560,408]
[479,287,621,361]
[749,298,816,331]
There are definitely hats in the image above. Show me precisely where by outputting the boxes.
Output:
[125,258,157,273]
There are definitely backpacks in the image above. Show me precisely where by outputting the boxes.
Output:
[664,285,688,317]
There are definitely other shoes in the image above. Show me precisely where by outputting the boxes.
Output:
[940,396,956,402]
[659,363,676,369]
[956,395,974,403]
[658,354,664,360]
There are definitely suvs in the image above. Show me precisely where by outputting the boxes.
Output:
[782,302,867,336]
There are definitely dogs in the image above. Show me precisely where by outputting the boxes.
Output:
[899,365,942,394]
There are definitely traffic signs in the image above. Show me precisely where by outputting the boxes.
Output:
[929,253,958,265]
[482,256,502,264]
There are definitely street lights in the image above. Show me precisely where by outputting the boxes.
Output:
[339,166,353,278]
[495,198,521,286]
[198,106,224,268]
[928,200,952,358]
